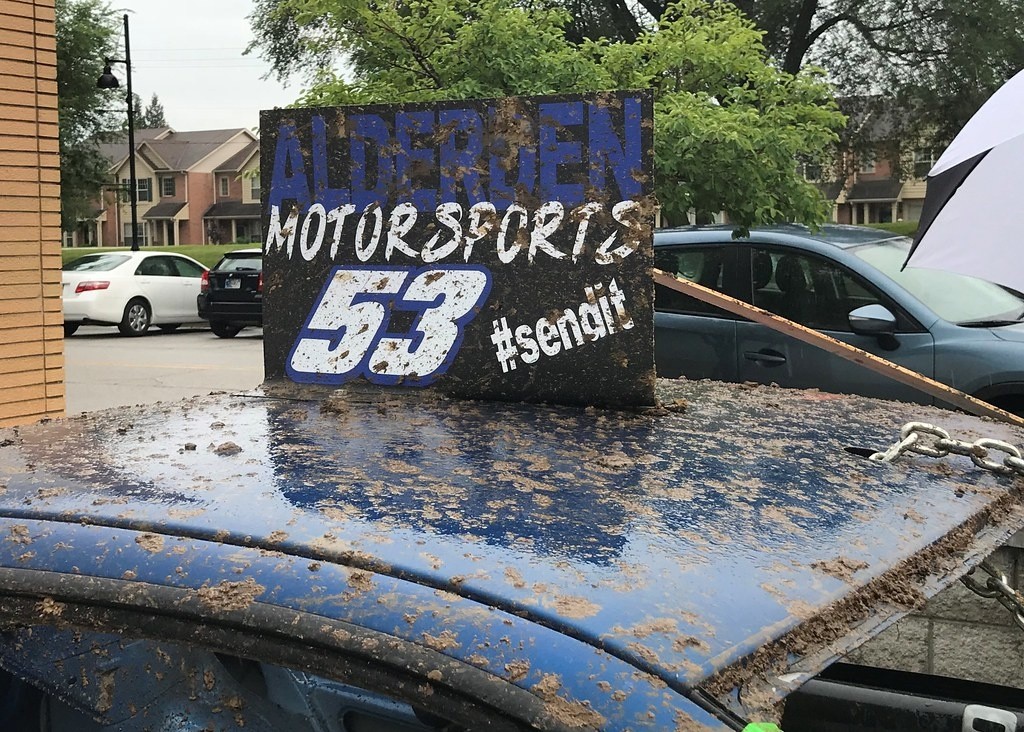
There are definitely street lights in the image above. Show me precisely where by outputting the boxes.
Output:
[98,12,142,251]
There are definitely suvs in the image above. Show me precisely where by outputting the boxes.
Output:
[193,248,264,342]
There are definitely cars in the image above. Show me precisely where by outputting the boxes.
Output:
[653,220,1024,415]
[0,379,1024,732]
[62,250,214,341]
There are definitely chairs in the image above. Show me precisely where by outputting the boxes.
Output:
[150,264,164,275]
[739,255,783,316]
[774,257,816,320]
